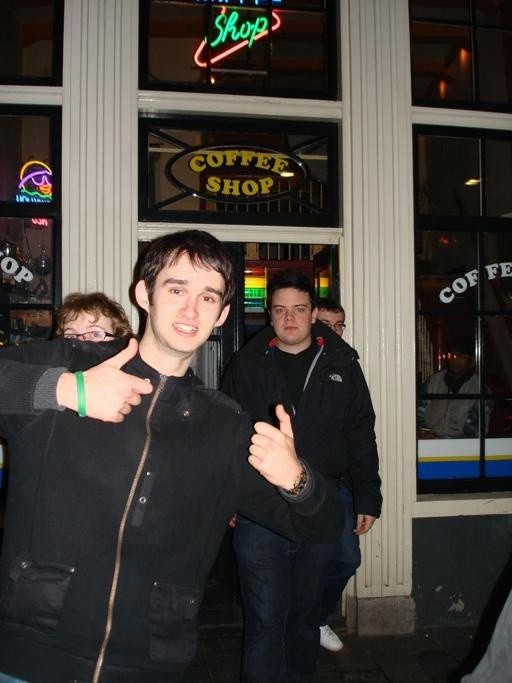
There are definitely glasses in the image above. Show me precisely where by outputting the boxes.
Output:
[64,330,122,343]
[320,319,346,332]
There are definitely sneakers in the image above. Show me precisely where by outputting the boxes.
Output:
[318,624,343,652]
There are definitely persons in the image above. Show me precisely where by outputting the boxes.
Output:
[416,336,494,439]
[217,270,383,681]
[2,230,347,682]
[54,291,132,343]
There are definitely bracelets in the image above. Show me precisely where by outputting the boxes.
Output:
[75,371,87,416]
[284,462,307,498]
